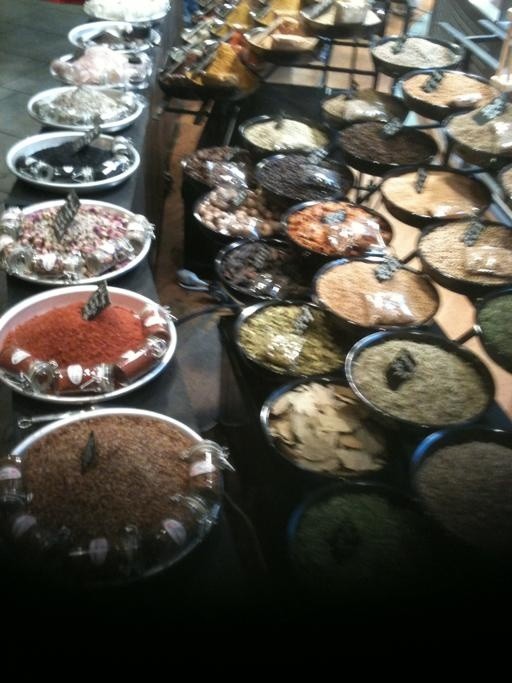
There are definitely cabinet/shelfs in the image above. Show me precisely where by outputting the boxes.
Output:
[156,0,511,683]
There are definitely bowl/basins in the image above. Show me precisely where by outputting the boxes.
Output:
[320,87,408,124]
[367,35,507,114]
[242,4,382,62]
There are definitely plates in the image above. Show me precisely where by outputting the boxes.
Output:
[261,374,407,481]
[0,406,226,586]
[444,104,512,159]
[7,131,141,188]
[50,46,155,91]
[282,196,392,256]
[250,153,357,204]
[0,195,155,286]
[238,113,335,156]
[338,120,442,170]
[416,217,511,294]
[180,146,263,197]
[314,255,440,331]
[234,302,347,380]
[27,86,144,132]
[84,0,170,22]
[192,188,288,242]
[409,423,512,556]
[215,241,320,308]
[290,481,423,598]
[0,285,178,404]
[343,327,495,427]
[374,163,494,225]
[71,21,162,52]
